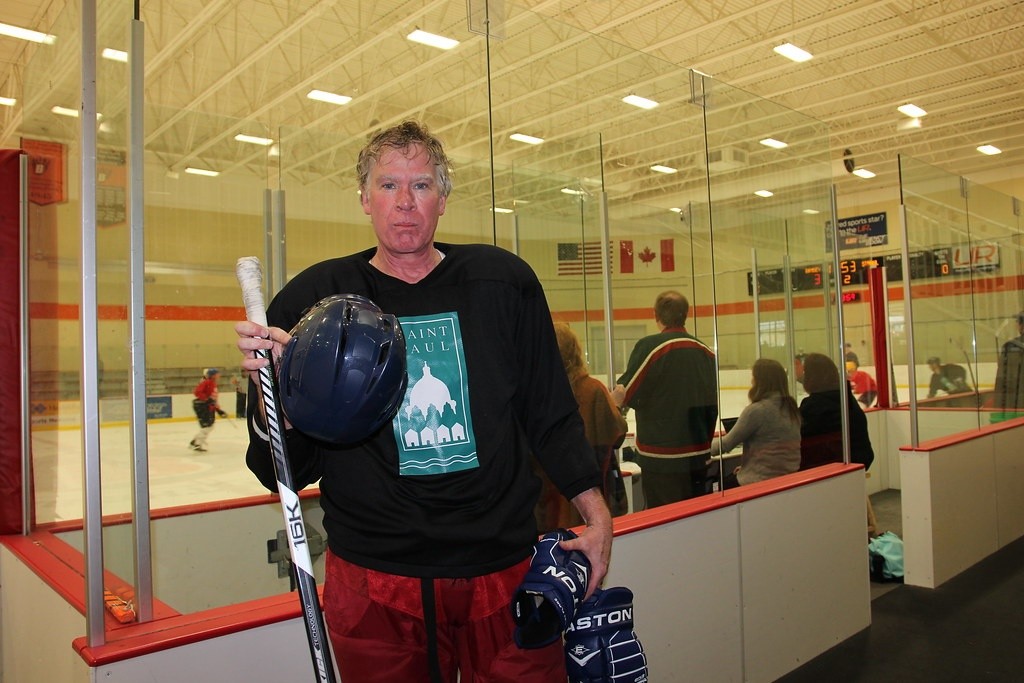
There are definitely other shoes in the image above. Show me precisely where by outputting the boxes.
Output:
[190,440,207,452]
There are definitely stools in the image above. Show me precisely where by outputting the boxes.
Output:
[866,471,880,536]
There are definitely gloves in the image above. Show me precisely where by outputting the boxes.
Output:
[511,528,592,651]
[565,588,648,683]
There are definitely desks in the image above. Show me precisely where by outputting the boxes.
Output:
[708,447,743,493]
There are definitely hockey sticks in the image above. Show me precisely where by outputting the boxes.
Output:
[214,404,237,429]
[233,254,337,683]
[949,336,977,396]
[994,319,1010,361]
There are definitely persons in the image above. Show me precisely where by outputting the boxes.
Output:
[791,351,875,469]
[840,343,859,371]
[617,289,718,508]
[710,359,801,490]
[988,309,1024,427]
[189,369,221,452]
[535,320,625,533]
[232,369,249,419]
[926,356,973,400]
[230,118,615,683]
[842,361,879,409]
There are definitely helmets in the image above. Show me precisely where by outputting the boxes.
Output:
[927,356,940,364]
[209,369,218,378]
[845,361,857,371]
[279,294,409,443]
